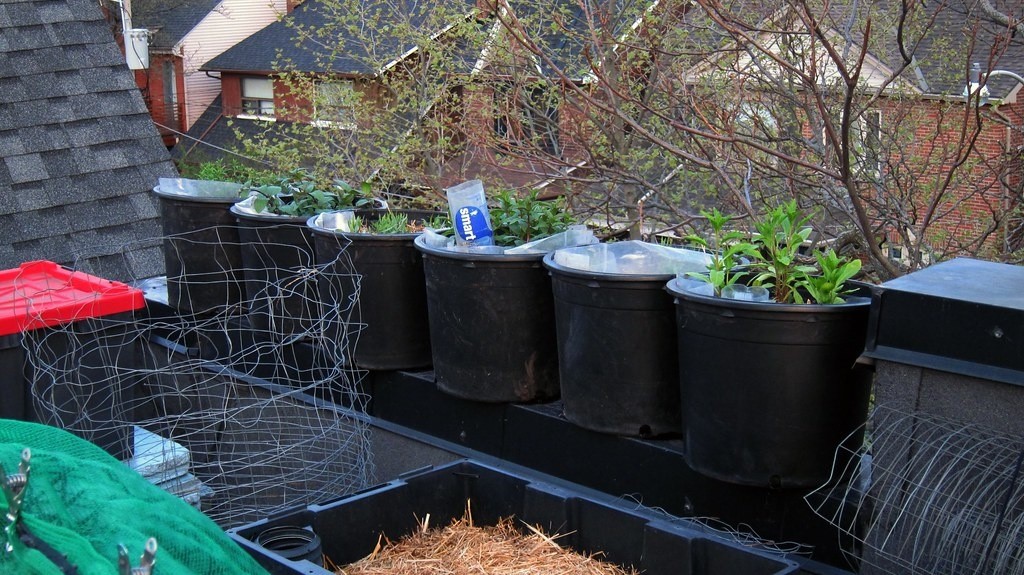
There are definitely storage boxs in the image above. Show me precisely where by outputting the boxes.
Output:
[224,458,799,575]
[0,259,145,461]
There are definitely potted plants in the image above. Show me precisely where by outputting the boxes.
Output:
[152,158,872,487]
